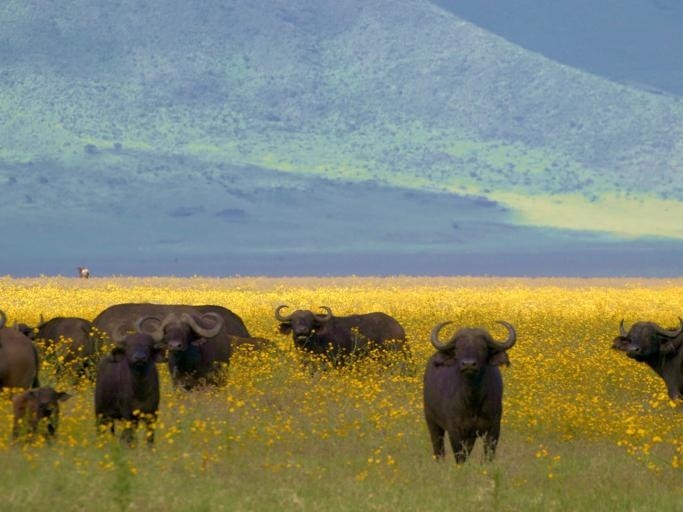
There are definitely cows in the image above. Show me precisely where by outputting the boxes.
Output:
[0,308,40,390]
[608,314,683,403]
[423,319,516,465]
[90,302,253,363]
[11,312,93,358]
[274,304,407,369]
[94,322,167,452]
[9,385,71,445]
[136,312,278,392]
